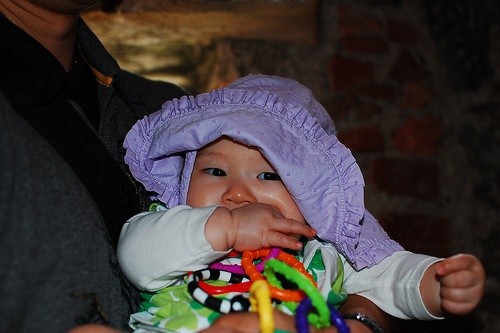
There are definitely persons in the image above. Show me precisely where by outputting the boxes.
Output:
[0,0,390,333]
[116,75,485,333]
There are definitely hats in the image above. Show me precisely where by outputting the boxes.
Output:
[123,74,406,271]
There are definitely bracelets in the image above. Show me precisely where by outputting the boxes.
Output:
[338,312,385,333]
